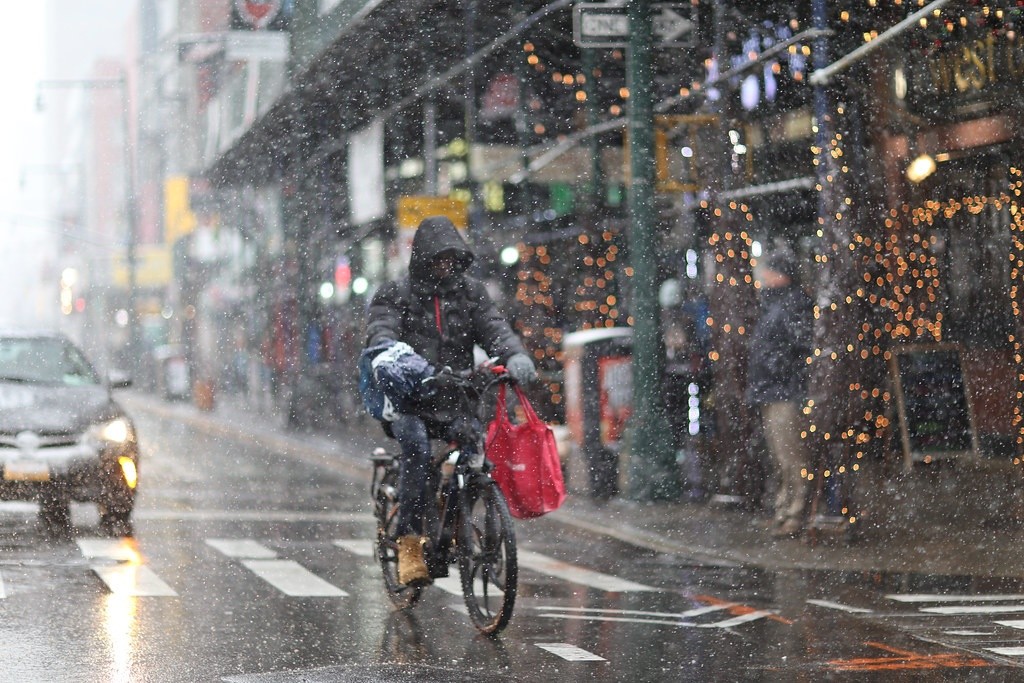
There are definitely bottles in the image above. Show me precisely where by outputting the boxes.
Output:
[435,449,463,506]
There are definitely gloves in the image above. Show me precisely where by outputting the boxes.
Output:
[506,352,537,385]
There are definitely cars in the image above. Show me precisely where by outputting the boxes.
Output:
[1,329,141,534]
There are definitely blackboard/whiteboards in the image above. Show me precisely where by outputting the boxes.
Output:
[886,342,979,462]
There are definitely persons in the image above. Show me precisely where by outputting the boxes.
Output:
[358,218,539,587]
[745,252,819,539]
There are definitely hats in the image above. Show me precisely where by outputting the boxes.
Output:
[766,254,793,277]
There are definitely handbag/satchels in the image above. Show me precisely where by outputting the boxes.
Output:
[484,366,565,519]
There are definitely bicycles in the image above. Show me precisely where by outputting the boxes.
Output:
[366,356,524,639]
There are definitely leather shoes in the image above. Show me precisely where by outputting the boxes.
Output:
[771,518,803,539]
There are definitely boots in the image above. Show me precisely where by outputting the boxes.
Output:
[395,533,429,586]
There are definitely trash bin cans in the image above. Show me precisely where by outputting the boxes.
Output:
[560,328,641,497]
